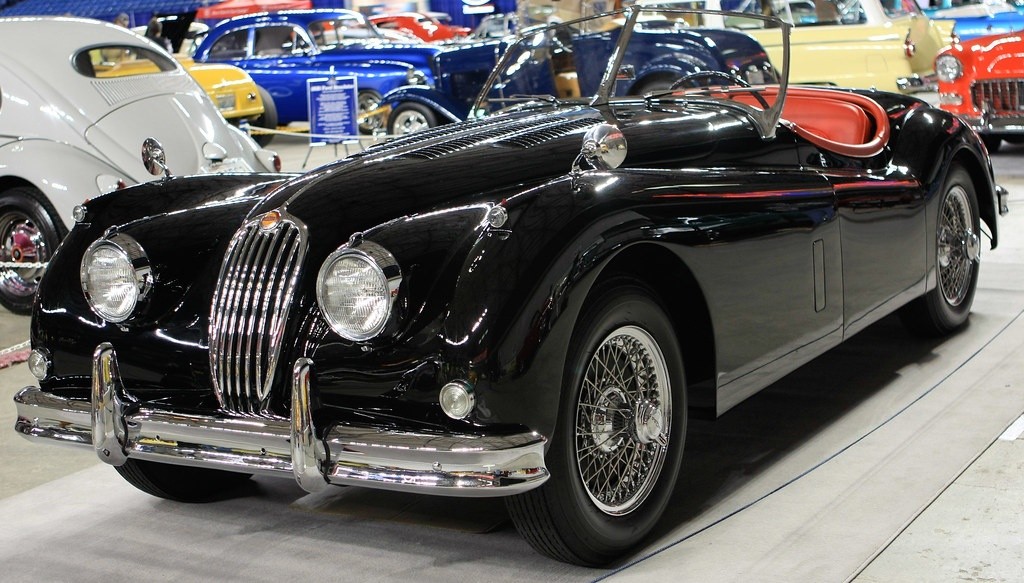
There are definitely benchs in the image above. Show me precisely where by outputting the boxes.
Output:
[709,94,869,144]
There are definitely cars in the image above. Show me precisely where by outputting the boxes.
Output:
[12,0,1013,570]
[893,0,1024,151]
[0,13,284,317]
[87,40,265,125]
[376,0,954,151]
[189,7,561,143]
